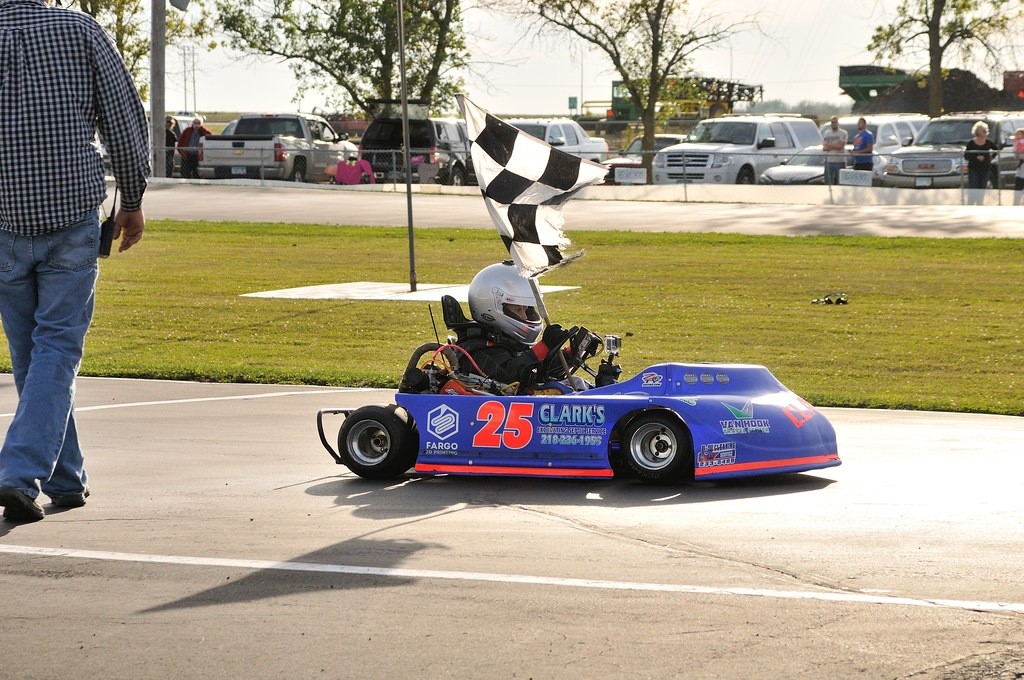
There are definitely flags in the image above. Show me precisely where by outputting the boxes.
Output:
[459,96,611,279]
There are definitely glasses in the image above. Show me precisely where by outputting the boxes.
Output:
[166,121,172,123]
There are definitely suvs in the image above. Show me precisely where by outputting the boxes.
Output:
[820,112,931,160]
[872,111,1024,189]
[356,117,477,186]
[651,113,824,184]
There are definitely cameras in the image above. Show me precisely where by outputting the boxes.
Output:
[605,336,620,354]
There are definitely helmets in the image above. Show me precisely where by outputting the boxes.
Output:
[468,260,543,343]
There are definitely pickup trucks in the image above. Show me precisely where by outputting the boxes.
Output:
[466,117,608,186]
[198,113,359,183]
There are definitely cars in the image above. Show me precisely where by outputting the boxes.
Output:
[592,133,696,185]
[758,145,887,185]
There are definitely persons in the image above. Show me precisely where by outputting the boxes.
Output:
[963,120,999,205]
[1013,129,1024,206]
[0,0,152,521]
[165,116,178,177]
[822,116,848,183]
[177,118,212,178]
[449,258,586,394]
[847,118,874,170]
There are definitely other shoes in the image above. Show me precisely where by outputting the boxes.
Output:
[0,487,45,519]
[52,489,91,507]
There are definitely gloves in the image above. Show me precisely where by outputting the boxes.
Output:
[542,324,570,350]
[571,334,598,356]
